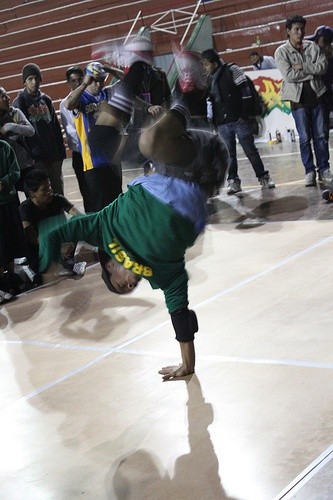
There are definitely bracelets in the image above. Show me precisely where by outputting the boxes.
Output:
[82,83,86,88]
[144,103,153,115]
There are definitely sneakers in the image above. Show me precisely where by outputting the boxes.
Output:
[89,41,154,74]
[175,50,207,93]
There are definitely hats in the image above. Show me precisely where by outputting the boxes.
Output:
[314,25,333,38]
[84,61,106,78]
[21,63,41,83]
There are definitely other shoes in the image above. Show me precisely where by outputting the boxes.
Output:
[258,174,274,188]
[321,189,333,201]
[226,177,241,194]
[304,170,315,186]
[71,262,86,276]
[318,169,333,183]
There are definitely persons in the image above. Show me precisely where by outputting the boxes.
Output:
[200,48,275,195]
[65,61,126,213]
[0,140,26,277]
[37,38,230,380]
[249,51,275,71]
[0,86,35,182]
[87,60,167,205]
[122,60,172,127]
[19,169,87,276]
[12,62,66,226]
[59,66,88,214]
[274,14,333,186]
[303,25,333,116]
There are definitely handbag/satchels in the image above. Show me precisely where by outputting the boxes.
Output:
[226,62,261,116]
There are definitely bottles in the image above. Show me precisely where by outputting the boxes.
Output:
[287,129,295,142]
[276,130,282,143]
